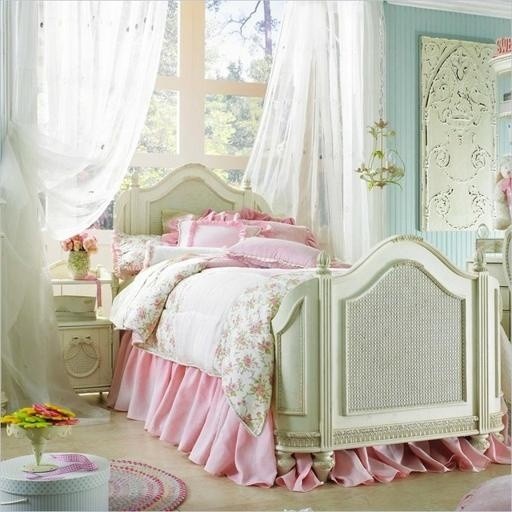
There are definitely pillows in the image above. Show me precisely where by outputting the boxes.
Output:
[161,208,352,268]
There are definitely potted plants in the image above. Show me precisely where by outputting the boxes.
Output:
[354,0,406,189]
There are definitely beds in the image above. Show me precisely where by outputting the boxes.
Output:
[103,161,512,492]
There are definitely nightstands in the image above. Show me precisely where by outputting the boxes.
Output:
[50,279,121,400]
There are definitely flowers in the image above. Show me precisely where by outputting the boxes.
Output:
[61,232,98,253]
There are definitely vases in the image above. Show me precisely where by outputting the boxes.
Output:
[68,251,91,279]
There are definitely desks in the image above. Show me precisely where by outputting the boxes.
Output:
[0,452,111,511]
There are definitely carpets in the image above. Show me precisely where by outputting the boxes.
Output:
[111,459,187,512]
